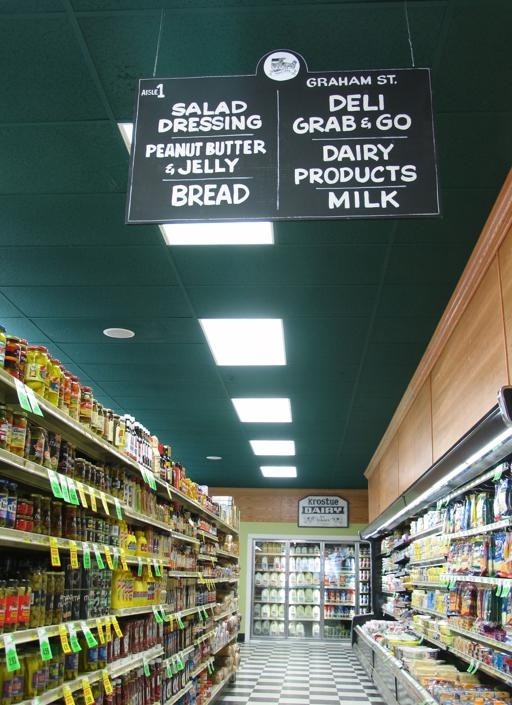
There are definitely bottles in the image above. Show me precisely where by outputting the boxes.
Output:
[112,413,182,705]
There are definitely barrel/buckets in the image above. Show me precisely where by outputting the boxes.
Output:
[252,540,322,639]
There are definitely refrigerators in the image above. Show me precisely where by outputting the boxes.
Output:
[252,539,371,641]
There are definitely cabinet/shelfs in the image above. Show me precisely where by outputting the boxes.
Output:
[244,532,370,643]
[0,317,241,704]
[349,164,512,703]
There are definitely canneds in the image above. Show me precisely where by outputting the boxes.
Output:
[0,326,120,705]
